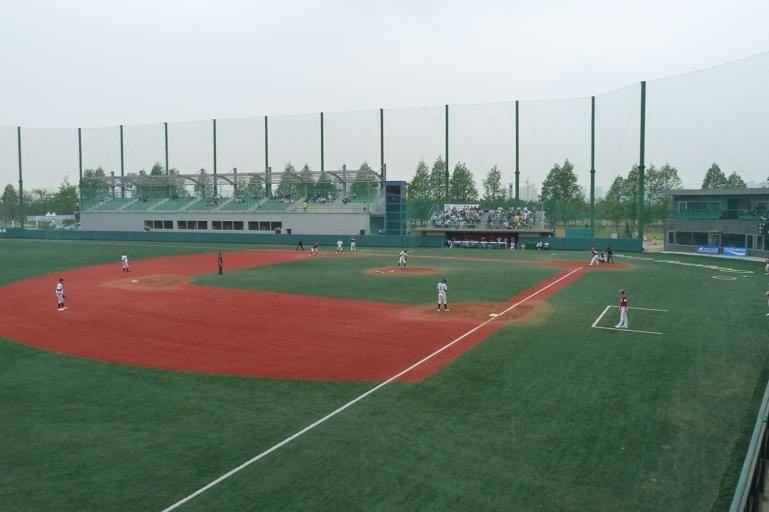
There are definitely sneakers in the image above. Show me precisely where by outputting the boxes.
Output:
[437,308,450,311]
[614,325,628,328]
[58,306,68,311]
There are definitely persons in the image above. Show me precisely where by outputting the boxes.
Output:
[435,277,451,312]
[121,251,129,273]
[432,204,535,230]
[588,245,615,265]
[398,249,408,268]
[537,241,542,249]
[510,240,515,250]
[55,278,68,311]
[614,289,629,329]
[336,240,344,254]
[296,240,304,251]
[218,253,223,274]
[275,189,352,213]
[350,236,356,251]
[310,239,320,255]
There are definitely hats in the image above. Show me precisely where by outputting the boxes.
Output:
[619,289,624,293]
[59,278,64,281]
[441,279,447,283]
